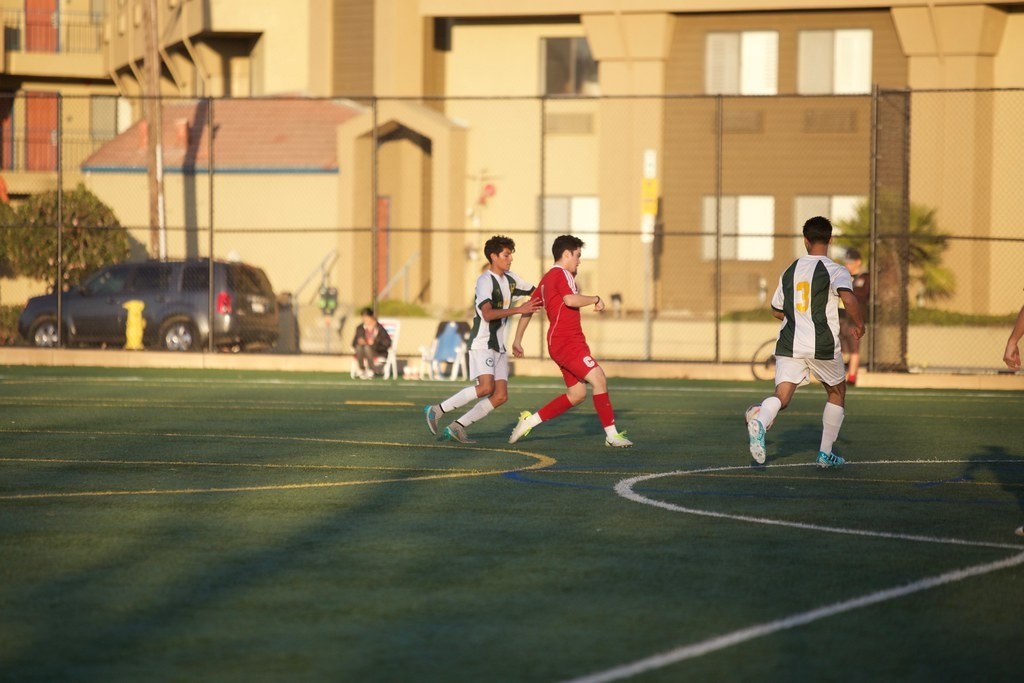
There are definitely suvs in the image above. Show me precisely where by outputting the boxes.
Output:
[17,256,280,353]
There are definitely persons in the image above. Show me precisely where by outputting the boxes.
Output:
[353,309,392,379]
[507,234,635,448]
[424,237,537,443]
[837,250,870,390]
[1003,306,1024,535]
[748,216,866,468]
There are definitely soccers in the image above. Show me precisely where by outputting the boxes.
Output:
[744,402,776,431]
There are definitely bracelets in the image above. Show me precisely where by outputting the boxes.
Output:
[594,295,601,305]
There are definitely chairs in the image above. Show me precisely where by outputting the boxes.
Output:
[351,318,401,379]
[418,321,470,381]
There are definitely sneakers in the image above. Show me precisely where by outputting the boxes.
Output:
[444,424,477,444]
[424,404,440,435]
[747,419,766,464]
[509,411,532,443]
[605,431,633,448]
[816,451,845,468]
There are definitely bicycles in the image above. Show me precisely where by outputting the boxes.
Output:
[751,337,849,382]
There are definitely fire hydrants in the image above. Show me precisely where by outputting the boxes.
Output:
[122,299,148,351]
